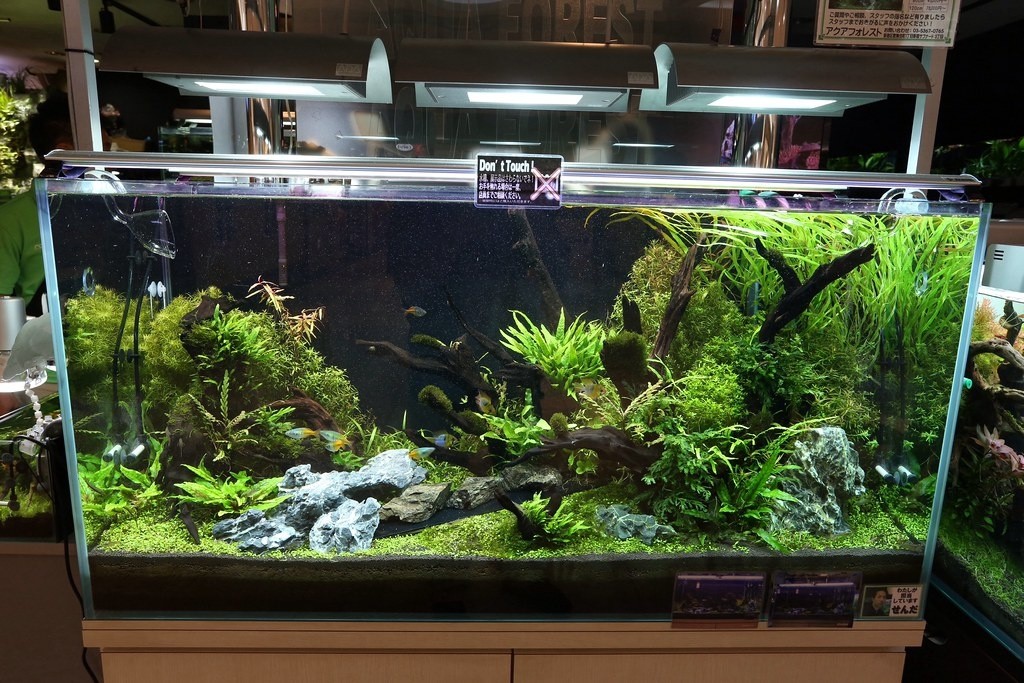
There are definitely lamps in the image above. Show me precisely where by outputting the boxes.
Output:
[87,0,394,107]
[652,0,933,118]
[395,0,659,113]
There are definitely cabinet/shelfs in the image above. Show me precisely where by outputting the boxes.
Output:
[79,619,928,683]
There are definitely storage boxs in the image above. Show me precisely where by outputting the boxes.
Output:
[929,217,1024,683]
[26,173,992,625]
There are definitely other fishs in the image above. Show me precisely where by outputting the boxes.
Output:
[284,427,352,453]
[410,434,458,460]
[475,392,495,415]
[578,380,606,398]
